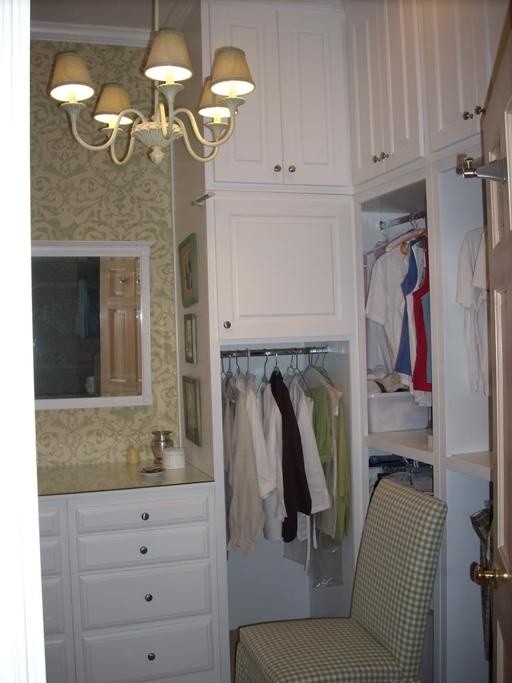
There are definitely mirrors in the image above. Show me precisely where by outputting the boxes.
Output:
[32,239,154,409]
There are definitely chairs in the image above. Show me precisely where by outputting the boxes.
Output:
[235,477,448,683]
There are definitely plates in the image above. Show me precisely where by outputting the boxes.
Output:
[136,465,165,478]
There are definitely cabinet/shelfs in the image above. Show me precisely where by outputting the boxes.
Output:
[39,482,223,683]
[170,0,512,683]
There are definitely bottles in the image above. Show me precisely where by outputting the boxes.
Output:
[149,430,175,466]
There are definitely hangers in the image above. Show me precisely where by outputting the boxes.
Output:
[217,347,331,401]
[364,210,428,257]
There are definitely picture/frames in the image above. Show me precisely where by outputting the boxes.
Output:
[177,232,201,447]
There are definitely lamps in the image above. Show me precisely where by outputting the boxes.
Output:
[46,0,258,167]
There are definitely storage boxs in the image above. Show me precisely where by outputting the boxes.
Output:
[367,390,431,432]
